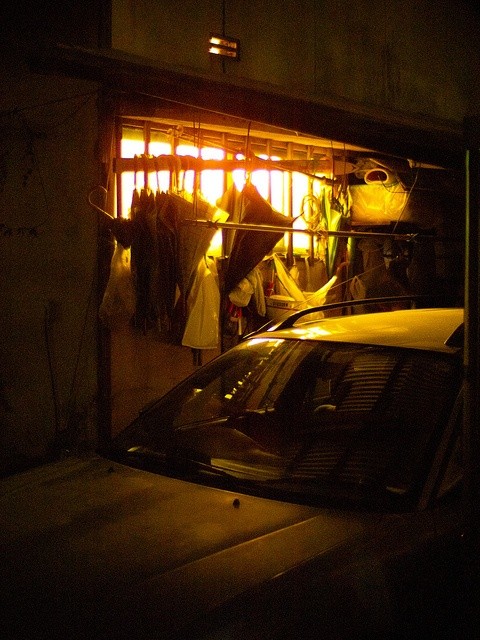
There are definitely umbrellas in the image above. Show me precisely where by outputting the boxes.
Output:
[317,155,353,282]
[215,119,306,307]
[86,146,229,337]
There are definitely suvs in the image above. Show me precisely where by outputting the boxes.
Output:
[2,297,478,640]
